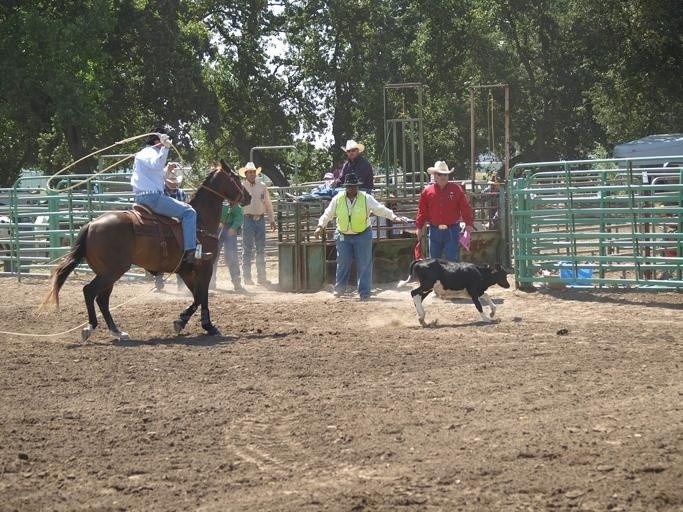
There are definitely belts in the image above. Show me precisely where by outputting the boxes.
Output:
[136,191,160,195]
[245,214,264,220]
[432,223,458,230]
[340,227,370,236]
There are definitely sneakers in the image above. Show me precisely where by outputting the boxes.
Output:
[361,292,370,301]
[152,277,272,292]
[334,289,345,296]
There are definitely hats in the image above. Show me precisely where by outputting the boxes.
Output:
[342,173,363,186]
[341,140,365,153]
[164,162,183,190]
[323,173,335,180]
[238,162,262,178]
[428,161,455,175]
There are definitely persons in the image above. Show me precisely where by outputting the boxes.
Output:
[237,161,277,286]
[152,160,189,293]
[209,195,247,294]
[309,171,334,191]
[130,130,214,267]
[415,160,474,258]
[284,138,374,205]
[312,173,410,299]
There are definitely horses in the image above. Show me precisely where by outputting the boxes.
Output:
[33,158,252,342]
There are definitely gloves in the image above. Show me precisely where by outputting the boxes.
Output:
[159,134,171,147]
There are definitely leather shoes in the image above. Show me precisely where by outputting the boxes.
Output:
[186,250,213,262]
[285,193,295,202]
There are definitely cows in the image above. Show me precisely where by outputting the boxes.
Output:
[396,257,510,328]
